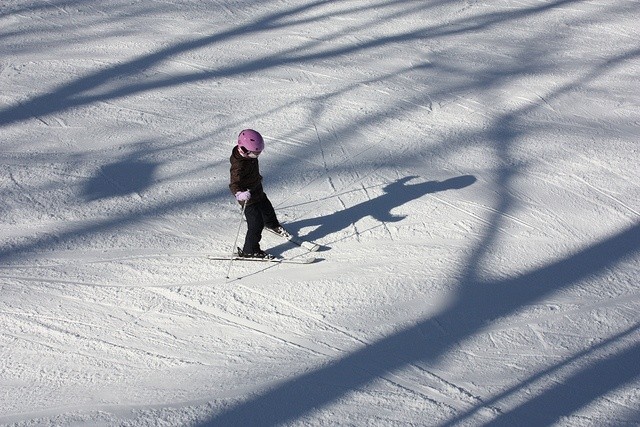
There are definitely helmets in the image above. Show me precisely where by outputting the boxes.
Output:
[237,129,264,158]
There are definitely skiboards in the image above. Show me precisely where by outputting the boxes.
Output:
[207,212,320,263]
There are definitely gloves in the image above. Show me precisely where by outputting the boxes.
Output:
[235,191,251,201]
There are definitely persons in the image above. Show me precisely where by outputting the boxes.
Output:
[229,128,282,258]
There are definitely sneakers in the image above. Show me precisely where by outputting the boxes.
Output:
[275,225,289,237]
[237,247,277,259]
